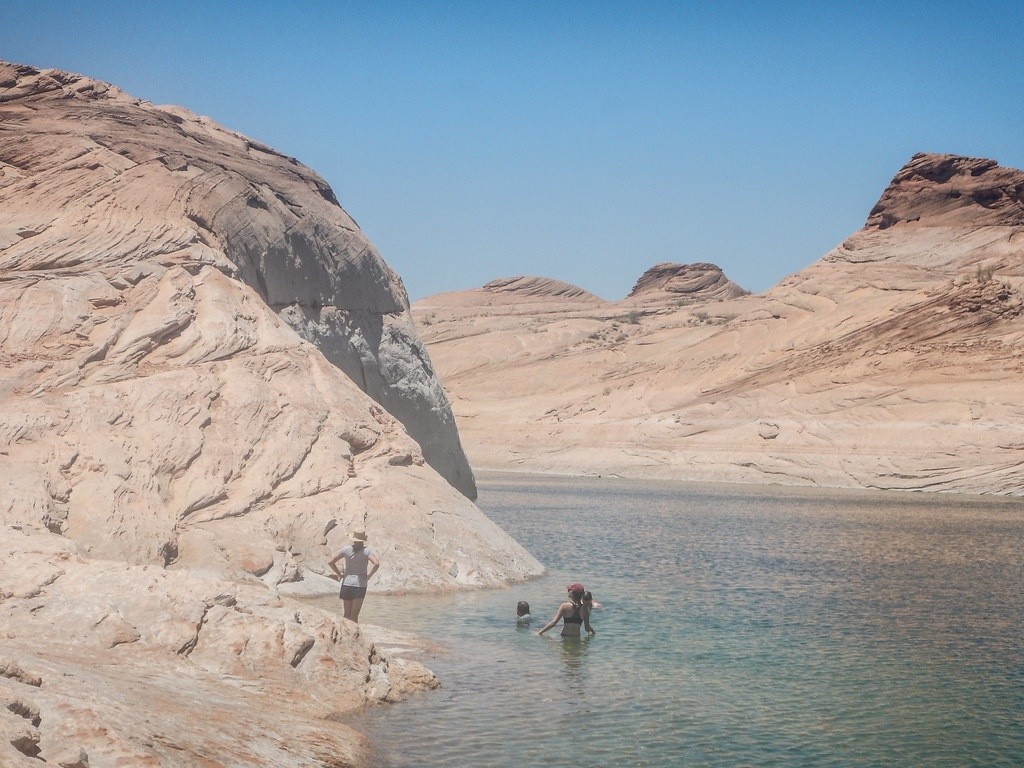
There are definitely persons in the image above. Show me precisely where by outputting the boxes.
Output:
[328,528,380,623]
[516,601,532,618]
[538,583,602,636]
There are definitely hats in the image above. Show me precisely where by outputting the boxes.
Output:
[517,601,529,611]
[566,584,584,592]
[349,528,367,542]
[582,591,591,600]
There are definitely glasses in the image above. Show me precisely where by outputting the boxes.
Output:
[567,589,571,592]
